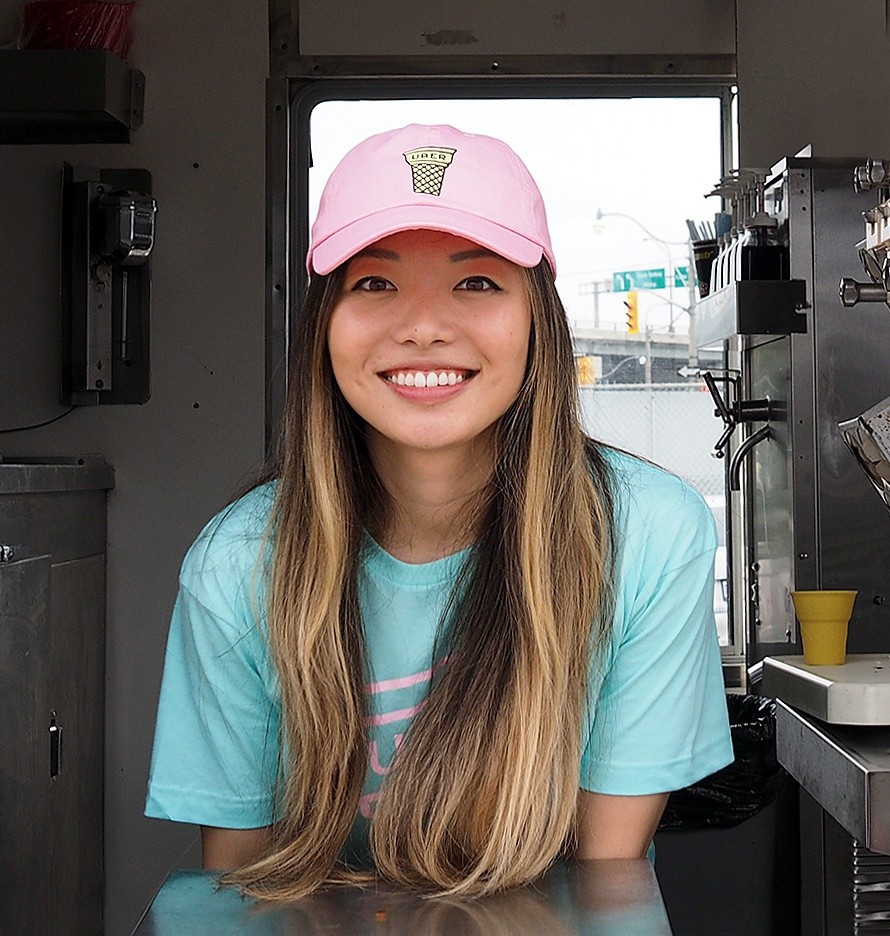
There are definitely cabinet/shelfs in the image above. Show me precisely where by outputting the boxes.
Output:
[0,456,116,936]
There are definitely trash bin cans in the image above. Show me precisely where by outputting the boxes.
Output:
[653,693,780,936]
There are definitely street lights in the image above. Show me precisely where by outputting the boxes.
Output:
[594,208,700,383]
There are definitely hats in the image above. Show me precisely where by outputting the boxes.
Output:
[305,123,557,288]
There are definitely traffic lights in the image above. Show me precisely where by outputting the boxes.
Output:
[623,290,639,335]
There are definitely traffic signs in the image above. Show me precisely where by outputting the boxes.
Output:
[613,268,665,292]
[676,367,725,382]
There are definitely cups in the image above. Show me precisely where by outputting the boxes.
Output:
[788,590,858,666]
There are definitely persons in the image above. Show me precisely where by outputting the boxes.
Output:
[143,124,736,872]
